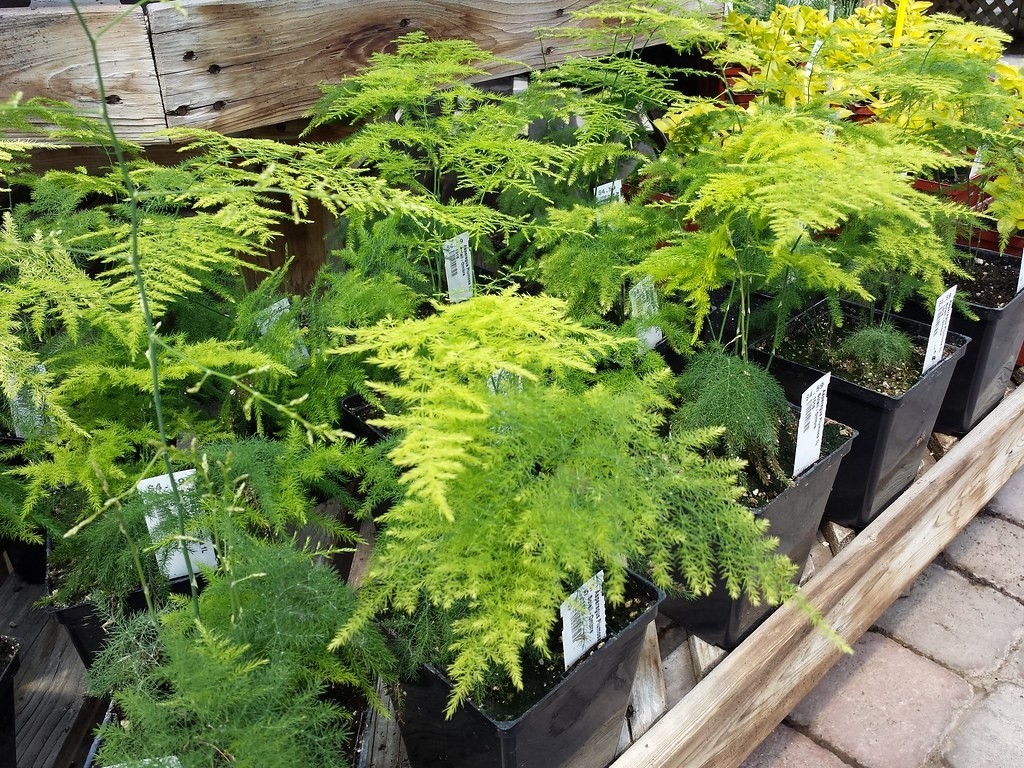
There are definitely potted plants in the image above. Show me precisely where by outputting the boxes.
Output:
[0,0,1024,767]
